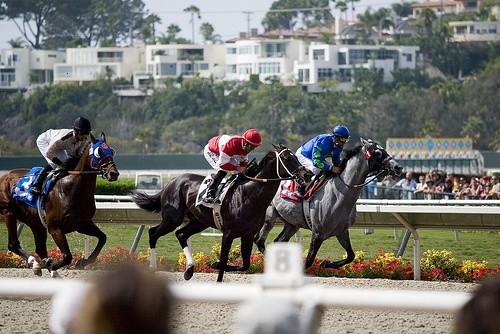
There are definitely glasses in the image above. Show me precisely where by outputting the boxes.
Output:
[339,138,349,142]
[77,131,90,136]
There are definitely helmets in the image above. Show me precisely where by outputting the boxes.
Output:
[333,125,350,138]
[241,128,261,146]
[72,117,91,132]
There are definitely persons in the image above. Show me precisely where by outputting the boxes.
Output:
[363,169,500,200]
[203,129,262,203]
[292,125,351,197]
[455,275,500,334]
[49,264,177,334]
[232,289,302,334]
[30,116,93,194]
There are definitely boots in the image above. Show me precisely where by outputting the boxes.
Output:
[294,171,314,197]
[202,170,227,201]
[30,167,47,193]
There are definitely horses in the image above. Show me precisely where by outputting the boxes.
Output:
[0,130,121,280]
[252,136,404,270]
[129,142,317,282]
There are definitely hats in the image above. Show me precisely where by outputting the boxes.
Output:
[426,179,434,184]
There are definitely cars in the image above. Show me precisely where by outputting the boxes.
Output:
[135,170,164,196]
